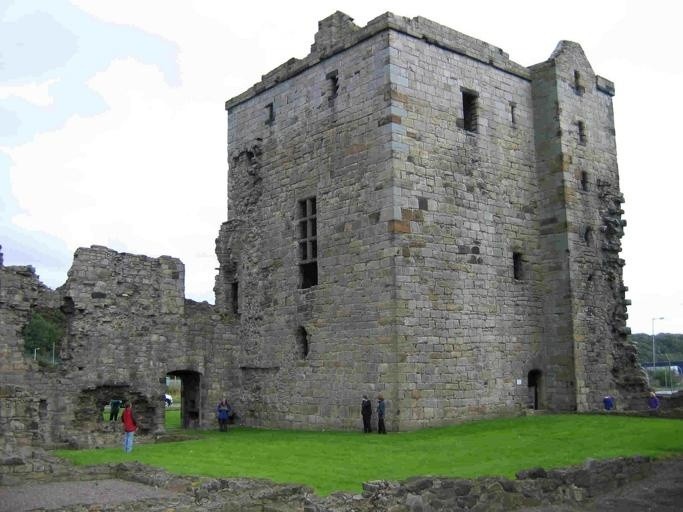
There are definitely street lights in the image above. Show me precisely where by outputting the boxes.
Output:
[652,317,664,372]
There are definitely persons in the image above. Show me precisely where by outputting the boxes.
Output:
[604,396,613,409]
[110,399,120,421]
[377,395,387,434]
[217,398,231,431]
[361,395,372,432]
[122,401,139,453]
[647,392,660,409]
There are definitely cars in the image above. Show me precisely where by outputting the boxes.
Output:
[165,394,173,407]
